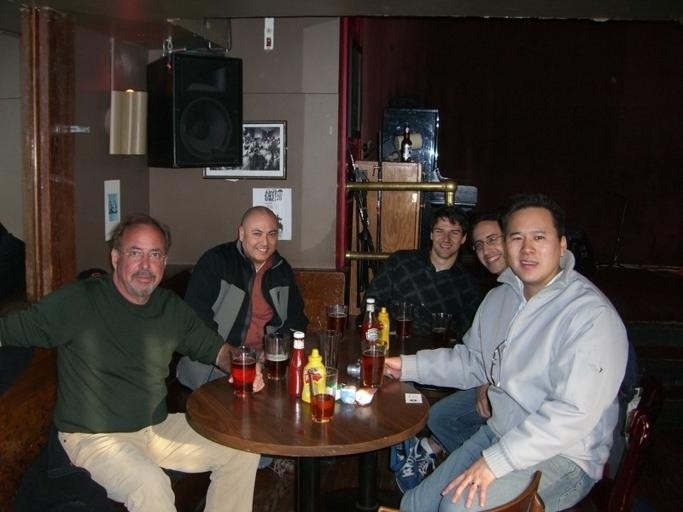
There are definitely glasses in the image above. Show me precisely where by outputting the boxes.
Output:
[115,249,168,262]
[473,235,506,251]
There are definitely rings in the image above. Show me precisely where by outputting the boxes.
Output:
[471,481,480,489]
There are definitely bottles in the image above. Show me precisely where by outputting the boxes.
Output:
[401,122,412,163]
[287,298,390,404]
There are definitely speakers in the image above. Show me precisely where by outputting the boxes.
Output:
[145,51,244,170]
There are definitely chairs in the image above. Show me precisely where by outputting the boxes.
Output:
[378,471,546,512]
[557,372,665,511]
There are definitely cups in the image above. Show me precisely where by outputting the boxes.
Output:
[229,302,452,423]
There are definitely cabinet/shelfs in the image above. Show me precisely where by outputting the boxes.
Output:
[349,159,422,316]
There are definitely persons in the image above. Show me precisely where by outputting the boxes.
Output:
[175,207,308,481]
[401,192,630,511]
[0,212,266,512]
[426,210,640,494]
[361,207,484,493]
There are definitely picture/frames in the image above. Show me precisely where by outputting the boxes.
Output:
[202,121,287,180]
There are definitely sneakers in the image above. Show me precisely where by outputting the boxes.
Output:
[416,440,437,484]
[394,435,420,494]
